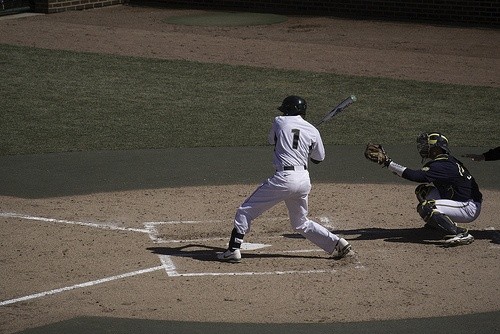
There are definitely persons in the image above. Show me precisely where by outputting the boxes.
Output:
[370,132,482,246]
[217,95,351,263]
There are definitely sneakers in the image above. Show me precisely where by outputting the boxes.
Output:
[444,231,474,247]
[216,248,242,260]
[333,237,351,258]
[416,225,441,234]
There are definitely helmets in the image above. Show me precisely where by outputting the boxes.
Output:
[277,95,306,116]
[427,132,451,154]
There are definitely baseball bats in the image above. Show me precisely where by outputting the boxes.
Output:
[314,95,357,129]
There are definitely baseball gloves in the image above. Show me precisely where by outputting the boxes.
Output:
[364,142,393,169]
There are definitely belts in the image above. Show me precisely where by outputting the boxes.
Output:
[283,165,307,171]
[473,199,482,203]
